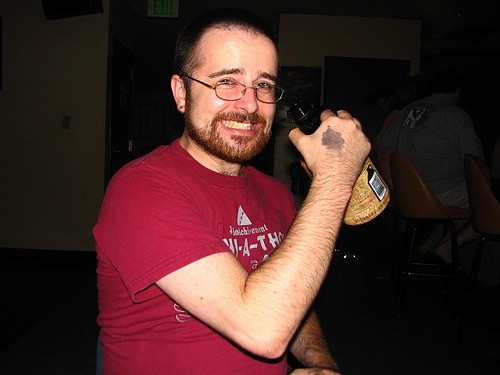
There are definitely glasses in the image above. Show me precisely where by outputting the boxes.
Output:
[180,71,286,104]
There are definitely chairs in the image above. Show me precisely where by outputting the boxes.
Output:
[378,148,500,344]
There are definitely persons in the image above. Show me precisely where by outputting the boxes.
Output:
[376,58,490,272]
[93,10,372,375]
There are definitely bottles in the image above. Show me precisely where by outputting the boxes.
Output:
[287,99,395,232]
